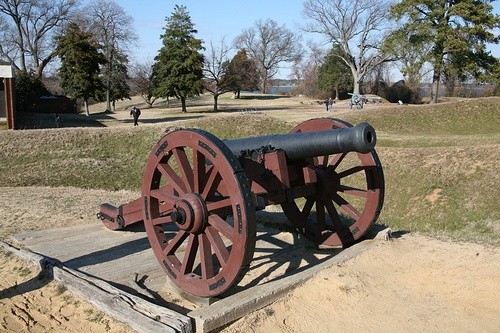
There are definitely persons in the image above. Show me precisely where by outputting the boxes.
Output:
[129,106,140,126]
